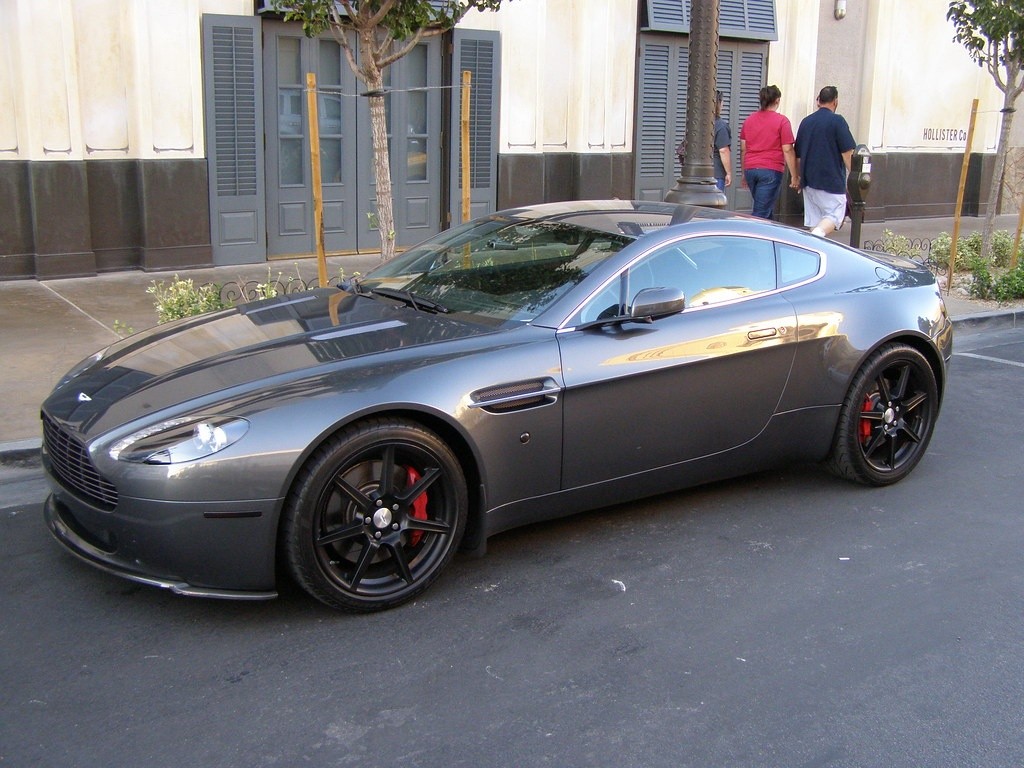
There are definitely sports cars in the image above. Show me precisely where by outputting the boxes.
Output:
[38,198,956,616]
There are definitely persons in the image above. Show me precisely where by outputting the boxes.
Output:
[794,86,856,237]
[740,85,800,219]
[713,89,732,194]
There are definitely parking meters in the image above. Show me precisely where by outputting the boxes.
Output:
[845,145,875,248]
